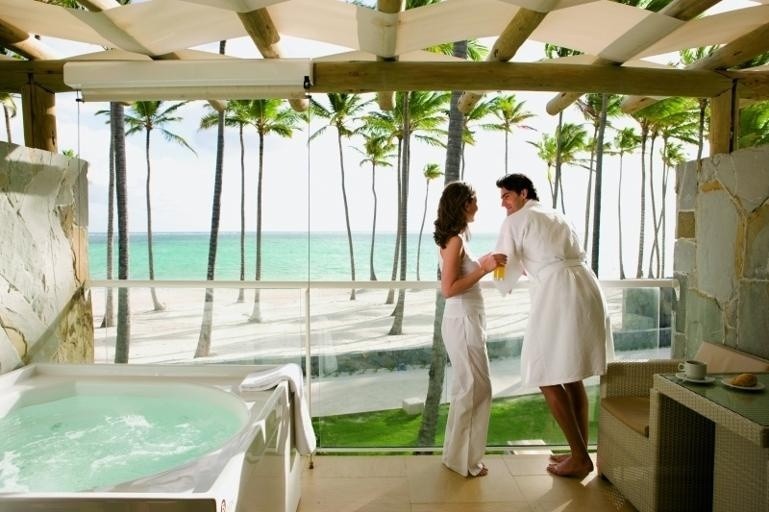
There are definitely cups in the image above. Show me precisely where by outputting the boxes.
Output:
[677,359,708,380]
[493,262,506,283]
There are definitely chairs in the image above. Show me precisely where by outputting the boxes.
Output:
[597,339,767,512]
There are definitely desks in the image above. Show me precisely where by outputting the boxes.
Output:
[654,366,769,512]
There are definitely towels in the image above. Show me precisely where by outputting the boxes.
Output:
[236,361,320,461]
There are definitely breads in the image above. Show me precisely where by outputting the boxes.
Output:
[731,374,757,386]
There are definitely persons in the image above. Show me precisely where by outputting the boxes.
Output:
[430,180,507,478]
[490,171,619,481]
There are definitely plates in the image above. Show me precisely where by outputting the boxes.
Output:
[720,377,766,391]
[675,372,717,384]
